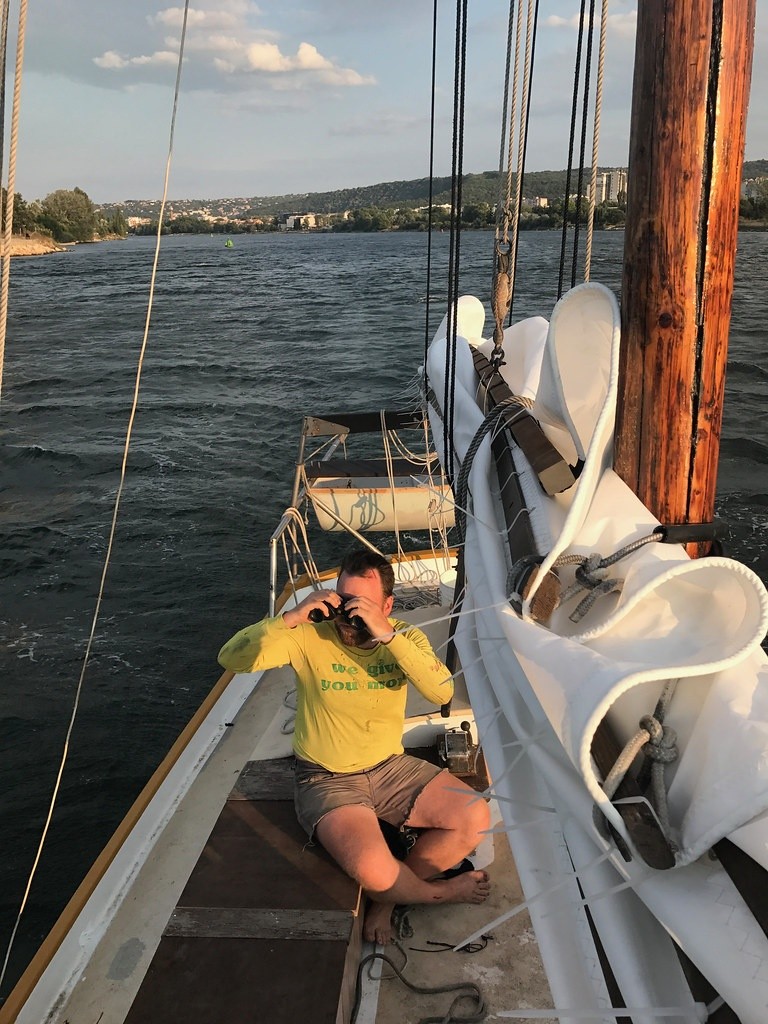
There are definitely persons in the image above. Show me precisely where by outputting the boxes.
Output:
[218,552,490,945]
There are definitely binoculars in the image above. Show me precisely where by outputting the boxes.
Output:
[308,595,365,630]
